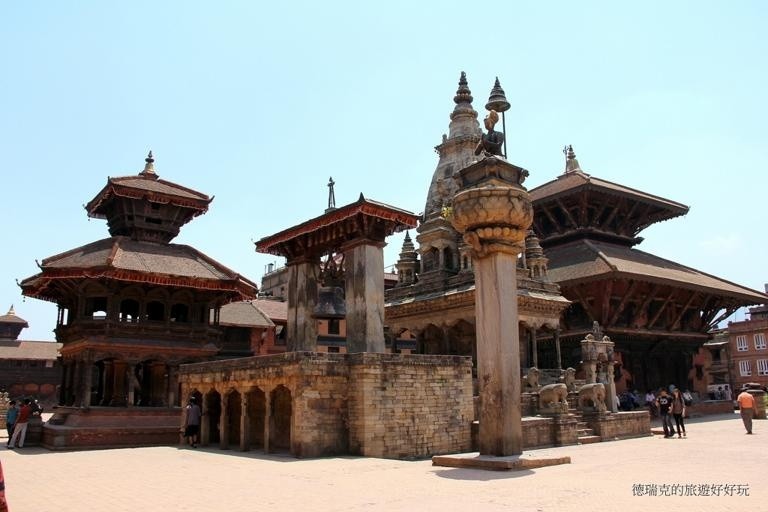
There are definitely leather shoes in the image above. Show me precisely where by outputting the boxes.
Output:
[746,431,752,434]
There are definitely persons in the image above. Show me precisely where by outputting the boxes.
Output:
[7,399,32,450]
[184,397,202,448]
[630,384,733,419]
[6,400,19,445]
[671,388,686,438]
[737,386,758,434]
[655,387,676,438]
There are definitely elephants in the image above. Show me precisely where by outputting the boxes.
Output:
[577,383,607,412]
[539,383,569,414]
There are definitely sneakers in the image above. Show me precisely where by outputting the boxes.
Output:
[191,441,197,448]
[664,430,686,438]
